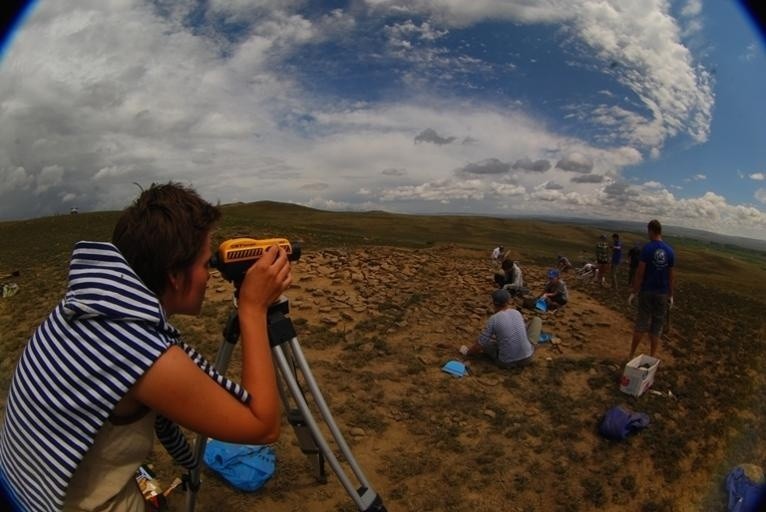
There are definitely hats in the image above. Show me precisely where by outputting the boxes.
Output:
[548,269,559,278]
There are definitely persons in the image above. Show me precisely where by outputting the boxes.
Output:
[1,182,292,512]
[537,269,569,308]
[626,220,675,360]
[596,233,622,292]
[491,244,523,296]
[558,256,571,272]
[577,261,595,273]
[627,241,641,285]
[459,289,542,369]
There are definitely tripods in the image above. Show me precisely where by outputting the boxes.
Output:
[182,294,389,512]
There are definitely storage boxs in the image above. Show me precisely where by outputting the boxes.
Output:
[619,353,661,397]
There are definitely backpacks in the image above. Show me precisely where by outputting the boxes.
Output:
[726,467,763,512]
[203,439,275,492]
